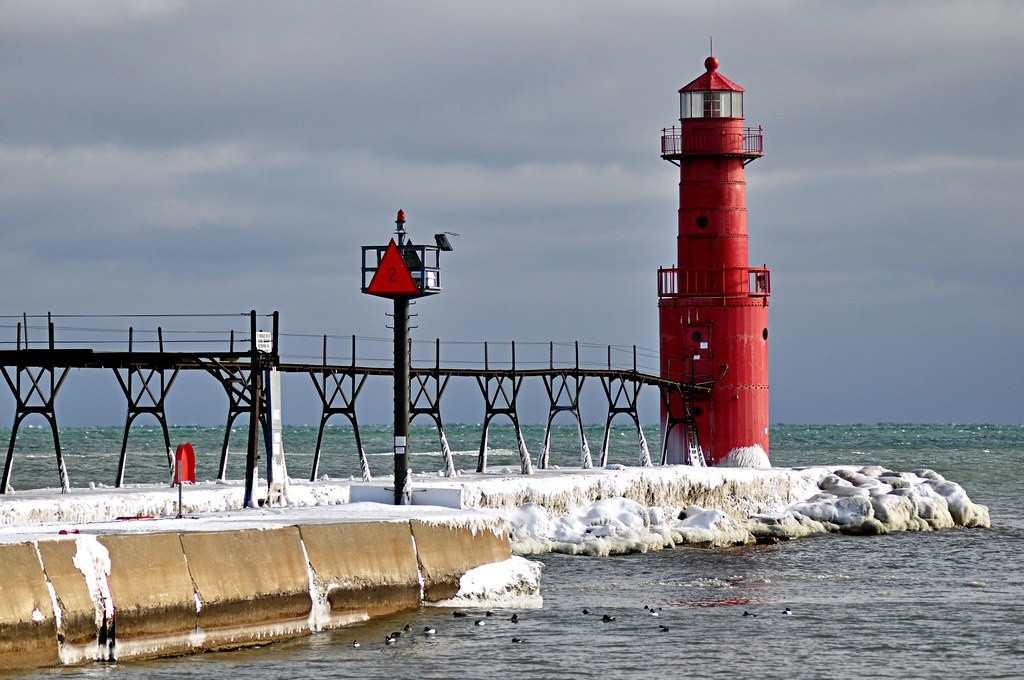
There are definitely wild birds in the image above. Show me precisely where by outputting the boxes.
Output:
[352,606,793,647]
